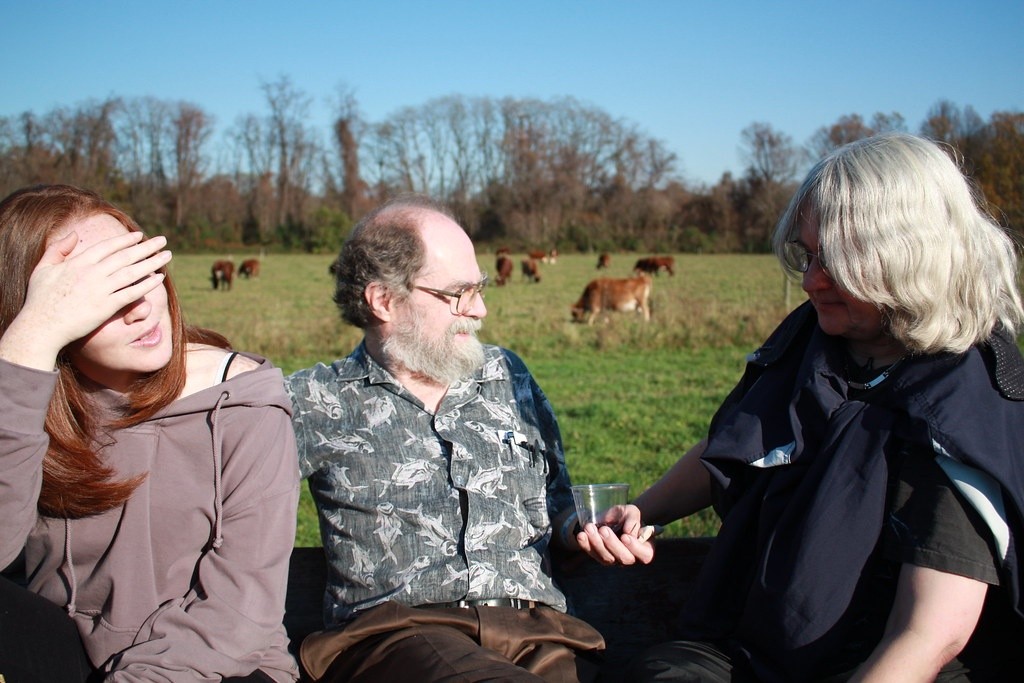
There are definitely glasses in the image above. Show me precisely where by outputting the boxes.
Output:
[781,241,835,279]
[408,272,488,315]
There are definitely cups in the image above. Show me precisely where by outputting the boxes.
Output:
[570,484,630,536]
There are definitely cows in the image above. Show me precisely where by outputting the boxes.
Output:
[596,252,612,269]
[496,247,512,285]
[239,257,260,277]
[634,255,677,276]
[526,248,560,264]
[207,260,235,292]
[572,279,651,325]
[521,257,542,282]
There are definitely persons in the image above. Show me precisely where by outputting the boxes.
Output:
[0,185,301,683]
[282,207,655,683]
[617,133,1024,683]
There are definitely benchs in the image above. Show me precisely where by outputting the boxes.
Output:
[285,538,714,650]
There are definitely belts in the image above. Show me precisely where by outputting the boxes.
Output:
[410,598,544,609]
